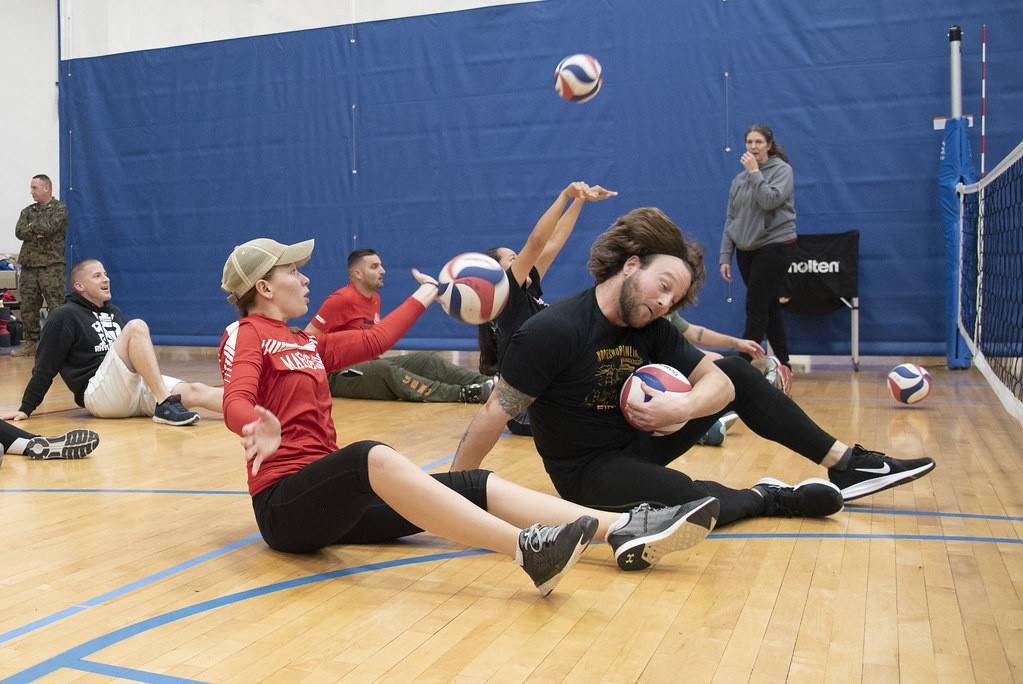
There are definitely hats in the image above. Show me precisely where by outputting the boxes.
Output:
[221,236,315,303]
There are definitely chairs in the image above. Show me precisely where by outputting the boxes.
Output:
[0,270,22,347]
[767,228,860,372]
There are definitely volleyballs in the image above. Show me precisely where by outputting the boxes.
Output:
[620,362,692,437]
[437,251,511,326]
[552,53,603,104]
[886,362,932,405]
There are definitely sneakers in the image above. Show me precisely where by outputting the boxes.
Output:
[605,494,722,573]
[825,443,936,502]
[460,375,499,405]
[27,429,100,461]
[773,367,793,398]
[11,339,38,355]
[152,394,203,427]
[519,515,599,598]
[719,409,738,430]
[694,421,727,446]
[751,478,844,521]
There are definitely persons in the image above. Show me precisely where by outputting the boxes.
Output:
[480,179,793,445]
[451,207,935,532]
[720,124,796,371]
[302,249,499,403]
[218,238,722,598]
[10,175,67,357]
[0,419,100,466]
[0,257,225,427]
